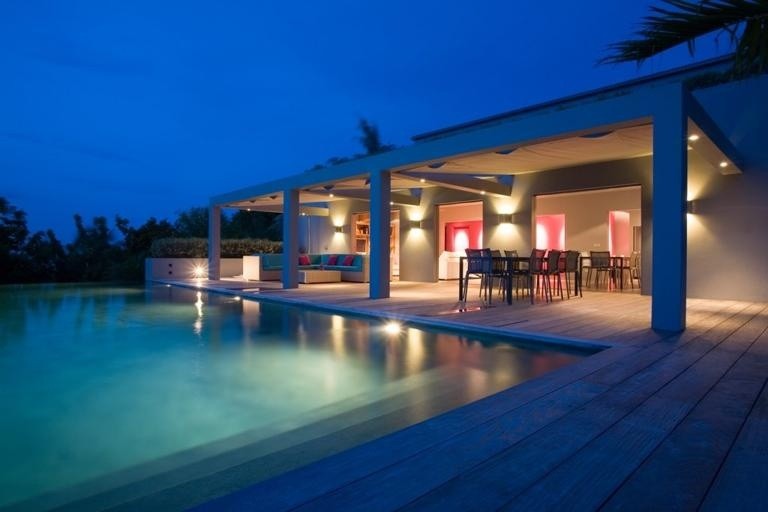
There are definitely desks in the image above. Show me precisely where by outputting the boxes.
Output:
[460,256,568,300]
[579,257,631,290]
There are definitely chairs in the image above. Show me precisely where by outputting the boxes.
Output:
[463,249,581,306]
[584,250,639,288]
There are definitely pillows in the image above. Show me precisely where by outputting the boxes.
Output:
[327,255,339,265]
[299,255,311,264]
[342,255,353,265]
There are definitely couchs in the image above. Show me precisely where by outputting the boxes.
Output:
[243,252,367,285]
